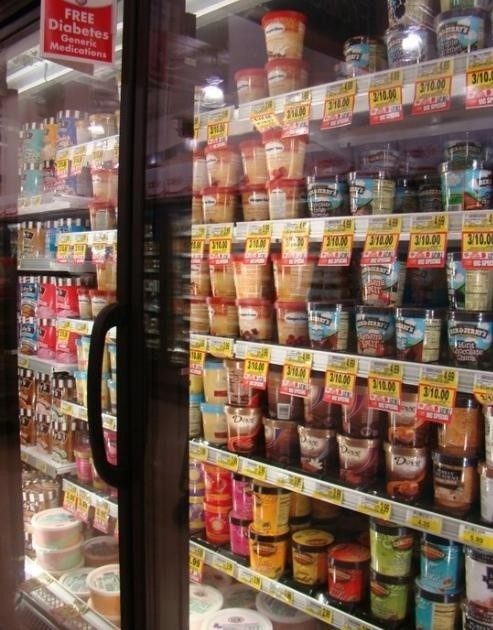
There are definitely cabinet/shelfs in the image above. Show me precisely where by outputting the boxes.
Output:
[20,1,492,628]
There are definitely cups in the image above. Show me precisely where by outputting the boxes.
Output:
[191,233,491,375]
[13,105,122,234]
[11,215,121,503]
[24,516,493,629]
[232,0,490,106]
[185,352,492,525]
[186,130,490,224]
